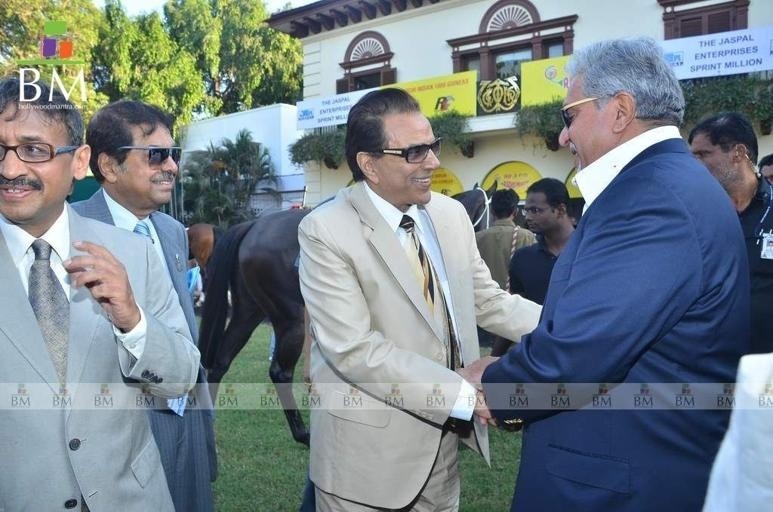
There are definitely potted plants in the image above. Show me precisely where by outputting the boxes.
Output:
[289,127,346,170]
[738,77,773,135]
[432,110,474,159]
[513,98,565,152]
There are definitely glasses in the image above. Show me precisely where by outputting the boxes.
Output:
[0,137,83,165]
[524,205,557,215]
[362,136,444,164]
[558,95,600,131]
[110,143,182,166]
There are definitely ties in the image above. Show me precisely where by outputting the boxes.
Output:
[397,214,459,373]
[23,237,73,393]
[130,219,158,248]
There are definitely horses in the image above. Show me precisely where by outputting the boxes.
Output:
[189,178,501,483]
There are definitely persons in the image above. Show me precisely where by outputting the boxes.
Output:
[0,78,201,512]
[69,100,218,512]
[299,39,772,512]
